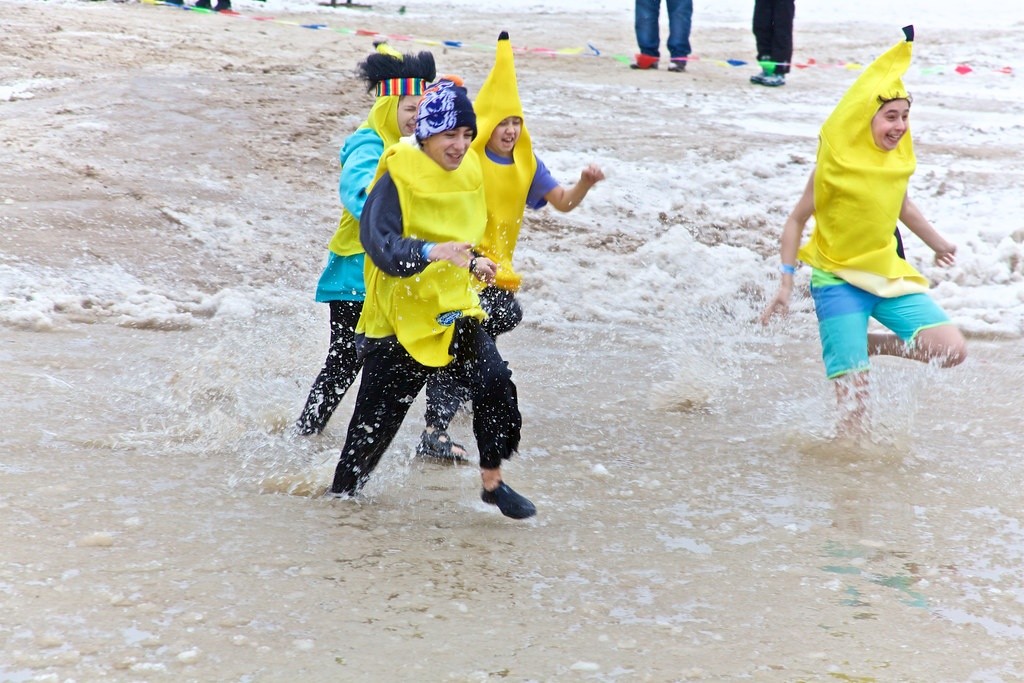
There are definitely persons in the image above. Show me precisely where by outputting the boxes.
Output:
[195,0,232,11]
[462,88,603,402]
[290,40,475,466]
[343,105,539,519]
[751,0,797,88]
[756,86,968,439]
[629,0,692,73]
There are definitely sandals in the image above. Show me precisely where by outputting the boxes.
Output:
[417,428,469,461]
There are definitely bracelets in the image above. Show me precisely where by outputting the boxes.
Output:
[468,258,478,273]
[778,260,797,275]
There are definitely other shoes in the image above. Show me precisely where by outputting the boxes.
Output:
[195,0,231,11]
[668,62,685,72]
[750,71,786,87]
[630,61,659,70]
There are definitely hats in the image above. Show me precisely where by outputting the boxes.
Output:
[414,76,478,148]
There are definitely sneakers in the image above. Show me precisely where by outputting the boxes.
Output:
[476,480,537,521]
